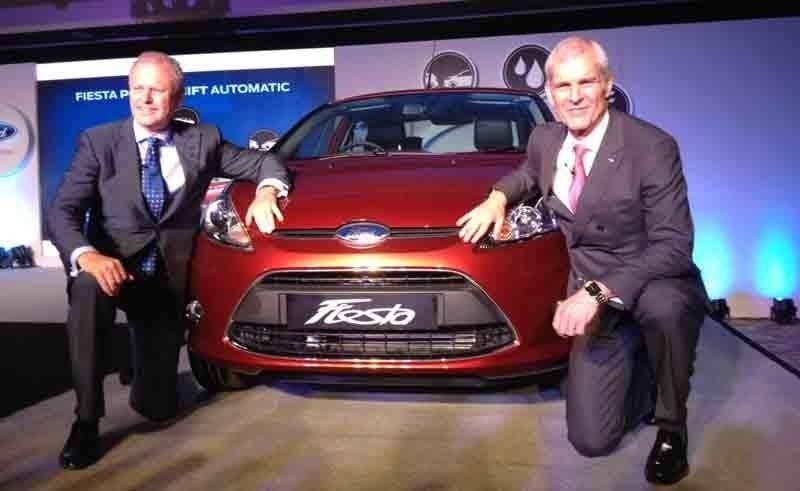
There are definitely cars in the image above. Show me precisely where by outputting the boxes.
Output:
[185,88,572,393]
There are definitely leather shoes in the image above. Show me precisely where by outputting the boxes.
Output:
[643,367,689,485]
[60,339,133,470]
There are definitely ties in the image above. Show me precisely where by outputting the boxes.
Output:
[568,144,589,217]
[137,137,165,276]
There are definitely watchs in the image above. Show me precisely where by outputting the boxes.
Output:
[583,278,608,305]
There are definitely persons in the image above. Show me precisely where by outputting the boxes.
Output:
[44,48,293,472]
[454,35,710,486]
